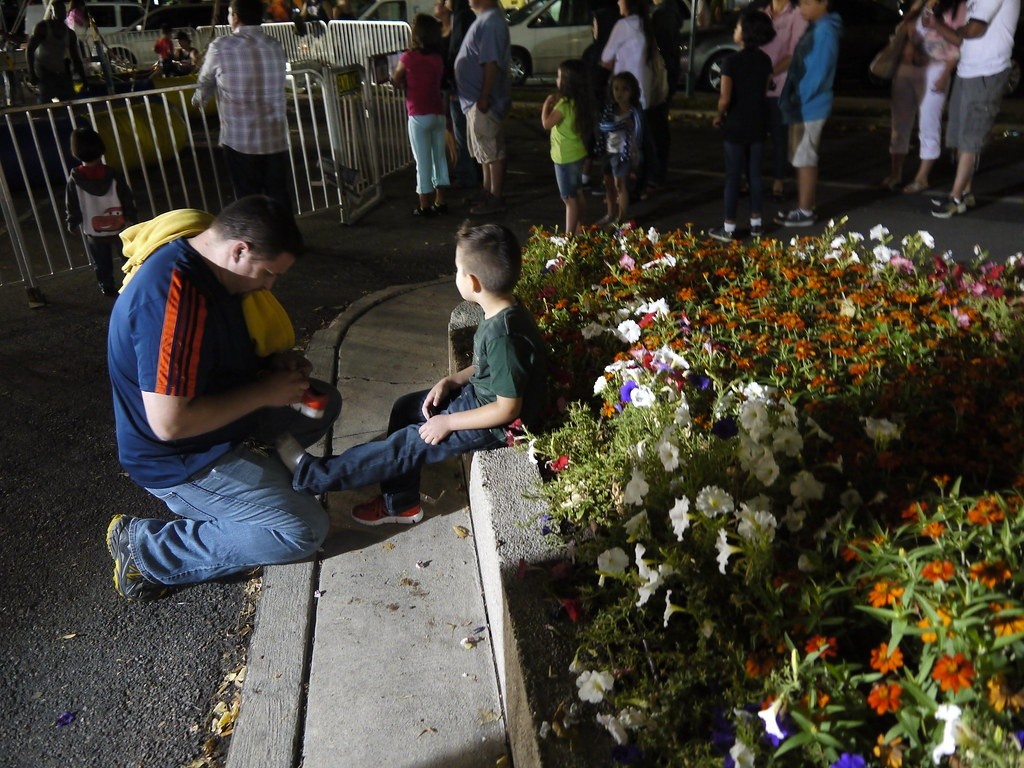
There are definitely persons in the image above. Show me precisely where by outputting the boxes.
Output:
[267,0,354,24]
[713,0,840,241]
[275,224,546,525]
[66,128,139,294]
[191,0,304,254]
[541,0,684,237]
[26,0,92,116]
[882,0,1021,217]
[108,195,344,600]
[145,27,200,83]
[391,0,513,214]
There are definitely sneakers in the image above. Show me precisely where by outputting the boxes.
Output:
[350,493,423,525]
[107,513,167,603]
[773,202,818,226]
[932,192,976,217]
[253,369,331,419]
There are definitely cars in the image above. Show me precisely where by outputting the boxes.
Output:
[61,1,149,61]
[91,3,241,80]
[336,0,440,41]
[504,0,711,88]
[675,0,904,96]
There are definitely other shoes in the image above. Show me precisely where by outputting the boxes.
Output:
[881,176,903,192]
[708,226,734,243]
[751,225,764,237]
[99,279,116,295]
[411,202,444,218]
[467,190,507,215]
[903,180,928,194]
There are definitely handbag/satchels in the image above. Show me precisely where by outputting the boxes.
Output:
[870,5,917,79]
